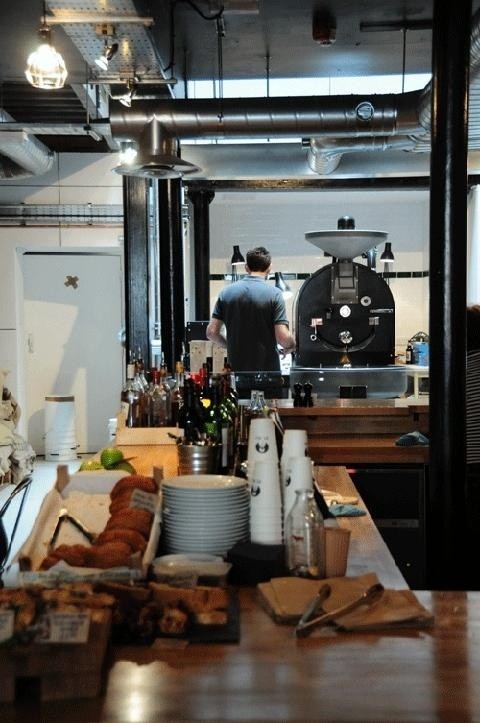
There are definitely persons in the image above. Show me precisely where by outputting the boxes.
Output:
[206,246,295,397]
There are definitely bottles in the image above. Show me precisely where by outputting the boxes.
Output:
[119,355,239,474]
[284,487,325,579]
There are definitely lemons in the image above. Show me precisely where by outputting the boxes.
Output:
[79,447,134,473]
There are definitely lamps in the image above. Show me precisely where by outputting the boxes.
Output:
[94,34,137,108]
[22,2,68,88]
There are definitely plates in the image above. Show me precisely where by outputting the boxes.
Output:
[160,474,251,558]
[153,552,234,578]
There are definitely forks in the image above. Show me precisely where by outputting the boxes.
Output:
[294,581,385,642]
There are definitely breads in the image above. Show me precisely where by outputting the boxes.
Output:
[36,474,158,572]
[0,579,230,633]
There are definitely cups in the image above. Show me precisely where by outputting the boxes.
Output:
[246,418,313,547]
